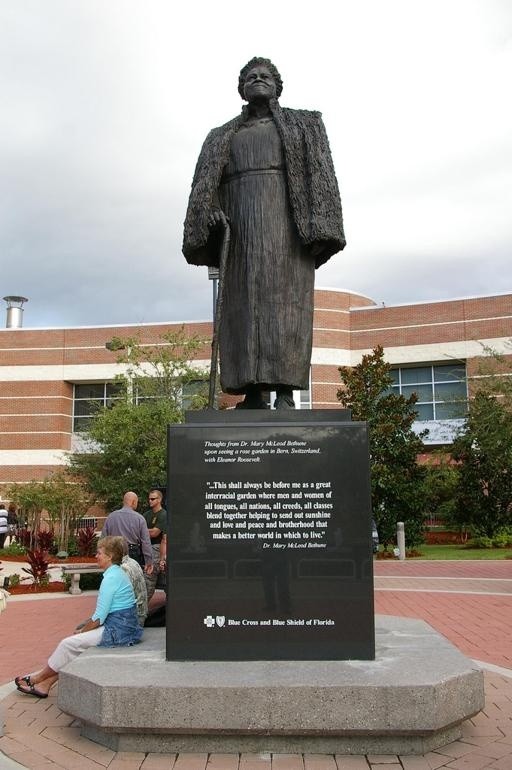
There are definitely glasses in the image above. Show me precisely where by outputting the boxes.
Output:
[148,498,158,501]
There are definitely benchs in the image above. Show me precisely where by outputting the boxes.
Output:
[62,563,105,595]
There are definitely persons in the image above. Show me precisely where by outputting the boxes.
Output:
[13,534,145,701]
[7,505,18,543]
[180,54,347,412]
[0,504,11,549]
[73,536,151,633]
[138,485,167,603]
[99,490,153,576]
[159,522,168,572]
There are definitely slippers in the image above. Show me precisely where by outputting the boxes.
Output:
[15,676,48,698]
